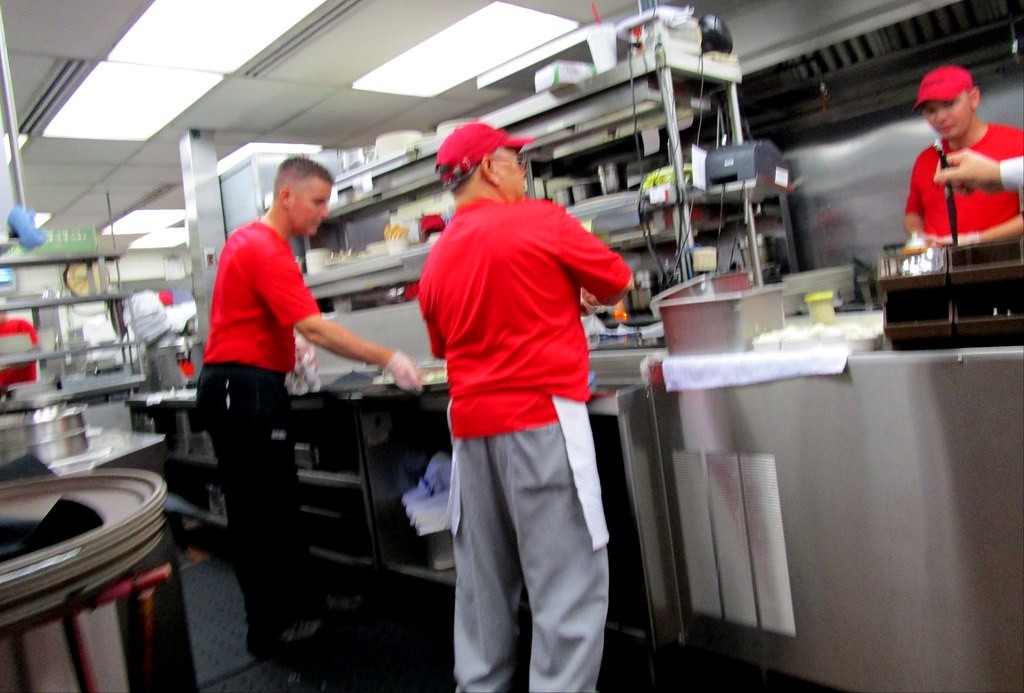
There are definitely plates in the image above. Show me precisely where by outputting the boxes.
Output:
[782,262,856,315]
[324,256,374,267]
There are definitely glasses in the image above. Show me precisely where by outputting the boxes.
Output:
[491,154,527,169]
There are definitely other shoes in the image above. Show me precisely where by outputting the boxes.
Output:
[313,593,364,619]
[247,618,323,654]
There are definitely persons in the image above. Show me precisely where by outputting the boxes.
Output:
[417,124,635,693]
[197,157,424,675]
[904,65,1024,249]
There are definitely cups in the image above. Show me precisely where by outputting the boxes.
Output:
[385,239,408,256]
[305,248,332,274]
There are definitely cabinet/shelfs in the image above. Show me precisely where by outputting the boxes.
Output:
[0,250,147,409]
[266,50,795,366]
[136,411,377,561]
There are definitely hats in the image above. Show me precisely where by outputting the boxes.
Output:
[912,64,974,115]
[435,123,534,183]
